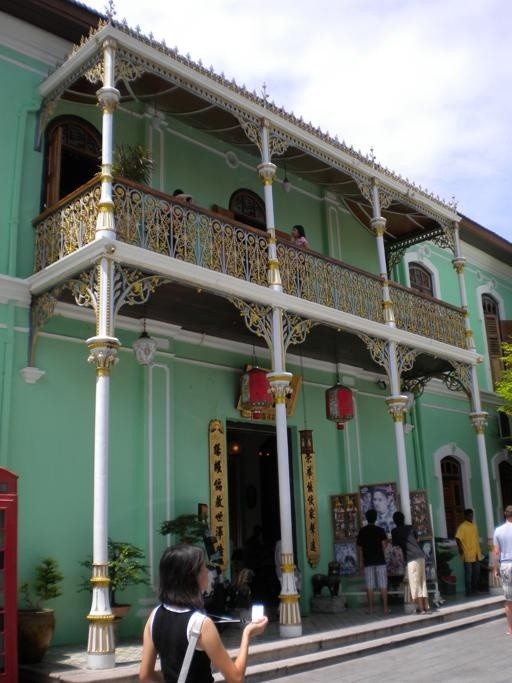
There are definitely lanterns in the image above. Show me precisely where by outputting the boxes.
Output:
[241,368,274,419]
[325,384,355,430]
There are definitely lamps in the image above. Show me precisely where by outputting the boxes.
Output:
[133,303,156,366]
[299,429,316,457]
[149,90,161,130]
[401,372,414,414]
[283,153,292,193]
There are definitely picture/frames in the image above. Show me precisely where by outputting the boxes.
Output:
[329,481,437,576]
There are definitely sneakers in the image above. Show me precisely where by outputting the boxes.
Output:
[466,592,480,596]
[412,607,431,614]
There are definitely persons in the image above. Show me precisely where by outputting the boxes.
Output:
[346,500,357,511]
[138,544,269,683]
[173,188,192,203]
[391,511,430,615]
[361,486,396,533]
[291,224,311,250]
[493,505,512,637]
[356,509,392,615]
[334,502,344,512]
[454,508,486,596]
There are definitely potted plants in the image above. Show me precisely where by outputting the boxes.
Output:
[94,142,157,244]
[78,537,151,620]
[18,558,65,665]
